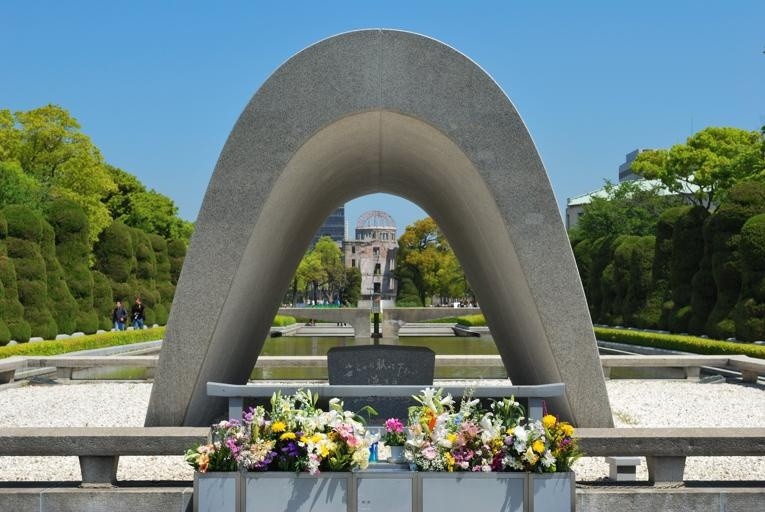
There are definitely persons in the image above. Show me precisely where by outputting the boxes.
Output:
[112,299,127,331]
[453,297,479,308]
[131,295,145,330]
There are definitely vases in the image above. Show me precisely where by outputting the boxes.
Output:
[382,387,573,473]
[191,387,377,473]
